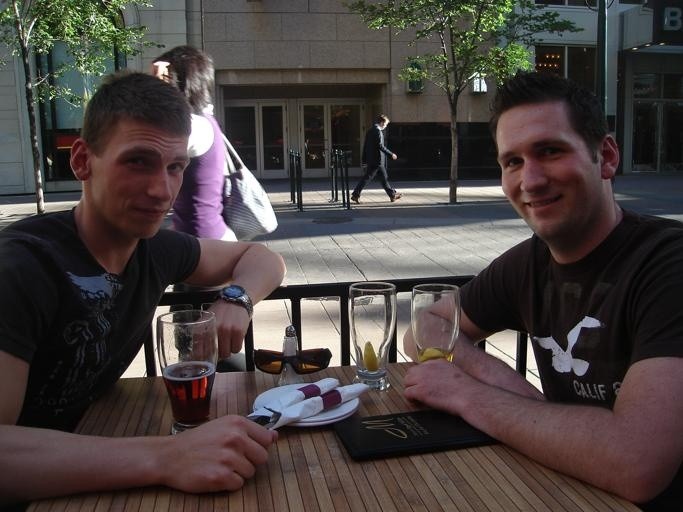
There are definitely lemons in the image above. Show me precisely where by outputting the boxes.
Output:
[364,342,377,371]
[419,348,444,362]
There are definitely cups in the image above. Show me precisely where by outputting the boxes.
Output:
[346,280,401,394]
[409,282,462,372]
[155,310,219,437]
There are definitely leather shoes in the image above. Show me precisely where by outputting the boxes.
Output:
[350,193,404,205]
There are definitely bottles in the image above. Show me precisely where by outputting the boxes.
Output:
[277,326,303,387]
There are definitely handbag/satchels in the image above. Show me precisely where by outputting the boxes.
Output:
[220,131,280,243]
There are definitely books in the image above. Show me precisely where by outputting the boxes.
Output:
[333,409,503,462]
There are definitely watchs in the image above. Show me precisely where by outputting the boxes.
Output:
[213,284,254,323]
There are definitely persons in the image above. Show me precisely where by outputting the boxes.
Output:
[151,45,247,373]
[0,72,285,512]
[398,73,682,512]
[351,114,402,205]
